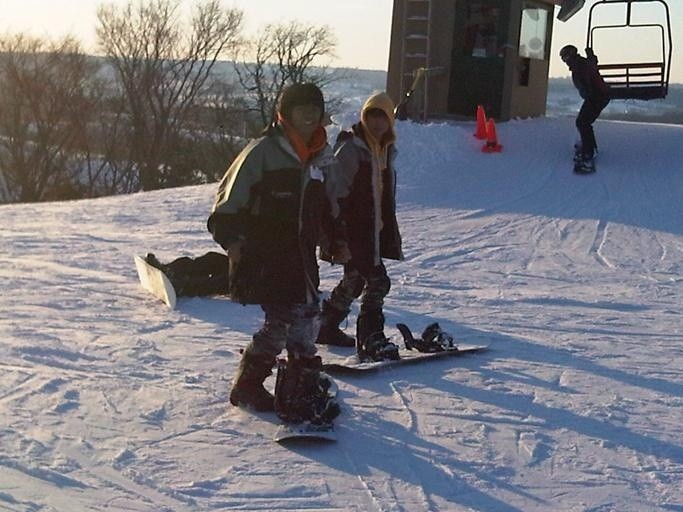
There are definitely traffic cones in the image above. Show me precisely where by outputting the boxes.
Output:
[473,105,503,153]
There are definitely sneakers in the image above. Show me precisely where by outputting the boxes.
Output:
[573,151,595,161]
[574,143,584,149]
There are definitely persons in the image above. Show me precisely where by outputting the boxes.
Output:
[145,250,232,299]
[203,82,347,428]
[559,44,613,174]
[317,88,406,359]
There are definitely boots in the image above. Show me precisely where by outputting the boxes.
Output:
[229,366,275,413]
[275,373,341,424]
[356,311,398,360]
[314,300,356,347]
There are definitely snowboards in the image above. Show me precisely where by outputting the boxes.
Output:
[133,252,177,309]
[323,343,488,372]
[573,140,595,174]
[274,371,339,442]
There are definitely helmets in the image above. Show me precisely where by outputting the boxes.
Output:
[560,45,576,61]
